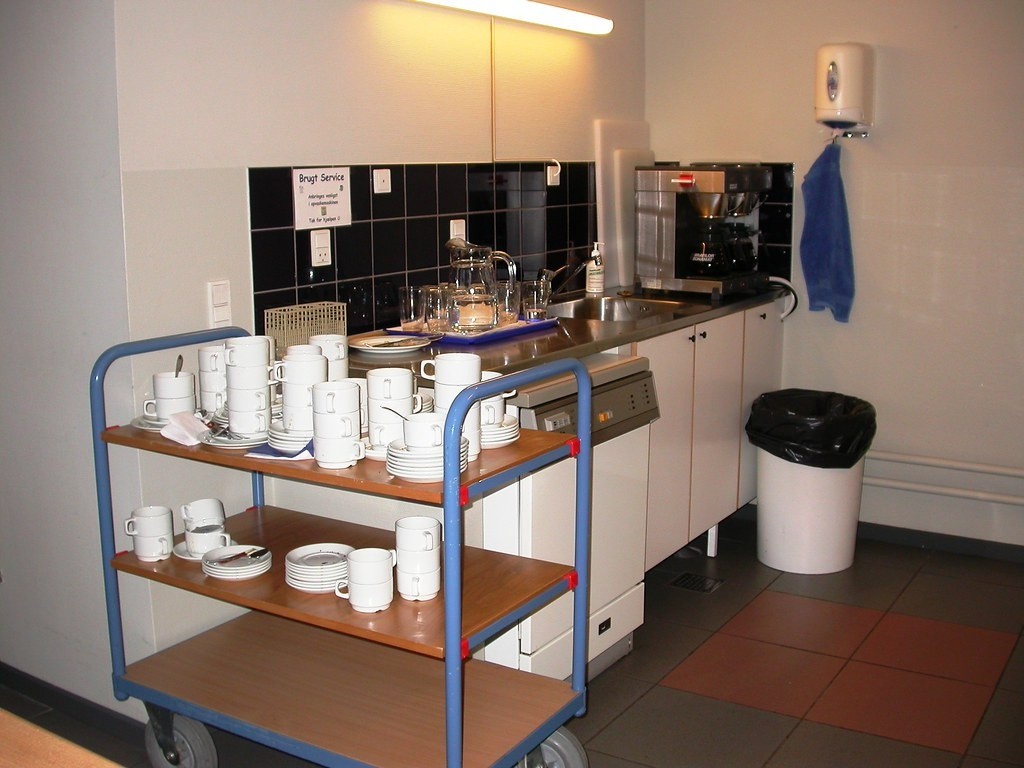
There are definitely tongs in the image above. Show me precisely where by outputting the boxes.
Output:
[373,334,444,347]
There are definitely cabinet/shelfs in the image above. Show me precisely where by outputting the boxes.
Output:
[637,302,785,583]
[90,327,592,768]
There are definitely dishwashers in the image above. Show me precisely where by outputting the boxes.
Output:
[485,370,661,681]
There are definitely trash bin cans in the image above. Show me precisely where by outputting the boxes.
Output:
[751,386,875,575]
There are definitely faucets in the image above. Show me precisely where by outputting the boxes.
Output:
[536,255,603,309]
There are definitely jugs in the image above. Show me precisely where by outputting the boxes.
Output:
[445,238,516,295]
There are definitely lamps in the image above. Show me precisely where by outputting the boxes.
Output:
[414,0,615,35]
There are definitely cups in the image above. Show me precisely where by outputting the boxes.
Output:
[481,371,517,428]
[335,516,441,613]
[399,281,546,331]
[274,333,413,469]
[125,497,231,562]
[225,336,281,433]
[421,353,481,462]
[403,413,445,450]
[143,346,227,421]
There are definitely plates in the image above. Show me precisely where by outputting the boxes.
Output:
[480,415,520,449]
[359,437,469,483]
[198,430,267,449]
[267,420,313,456]
[347,331,430,353]
[131,417,169,433]
[270,394,283,418]
[173,539,238,561]
[415,392,434,413]
[212,407,228,424]
[201,543,355,594]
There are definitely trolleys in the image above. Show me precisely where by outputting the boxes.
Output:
[90,327,590,768]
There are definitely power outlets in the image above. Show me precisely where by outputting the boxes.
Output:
[311,229,332,266]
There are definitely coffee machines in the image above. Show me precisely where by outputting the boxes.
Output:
[634,165,766,294]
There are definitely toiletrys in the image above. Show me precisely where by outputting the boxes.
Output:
[586,242,605,293]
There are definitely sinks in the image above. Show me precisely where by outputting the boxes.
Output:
[545,296,694,323]
